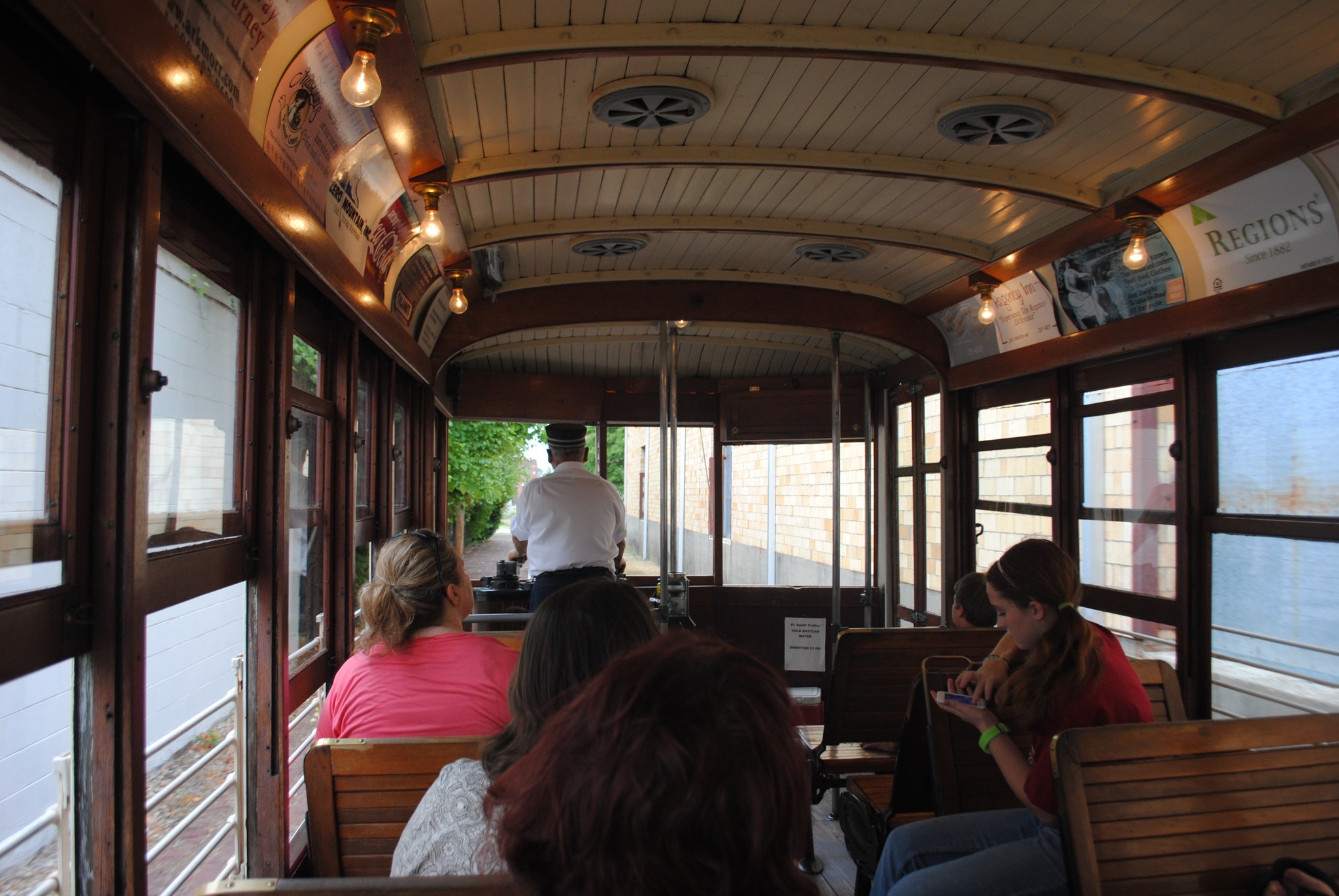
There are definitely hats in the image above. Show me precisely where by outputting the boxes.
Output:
[545,422,587,448]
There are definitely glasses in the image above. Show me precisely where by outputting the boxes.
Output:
[388,531,448,598]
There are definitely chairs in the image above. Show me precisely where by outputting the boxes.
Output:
[1048,711,1338,894]
[832,656,969,896]
[302,737,490,880]
[794,625,1008,876]
[921,654,1182,816]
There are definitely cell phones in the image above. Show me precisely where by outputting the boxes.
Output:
[937,690,987,710]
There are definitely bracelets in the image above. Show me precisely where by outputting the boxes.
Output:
[515,549,526,557]
[981,654,1010,671]
[978,722,1011,755]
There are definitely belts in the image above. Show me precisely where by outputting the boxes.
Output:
[536,567,613,578]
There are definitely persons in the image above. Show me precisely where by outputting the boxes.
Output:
[1060,256,1123,331]
[507,421,629,614]
[313,527,522,748]
[388,575,664,878]
[856,571,1001,776]
[477,629,827,896]
[868,537,1159,896]
